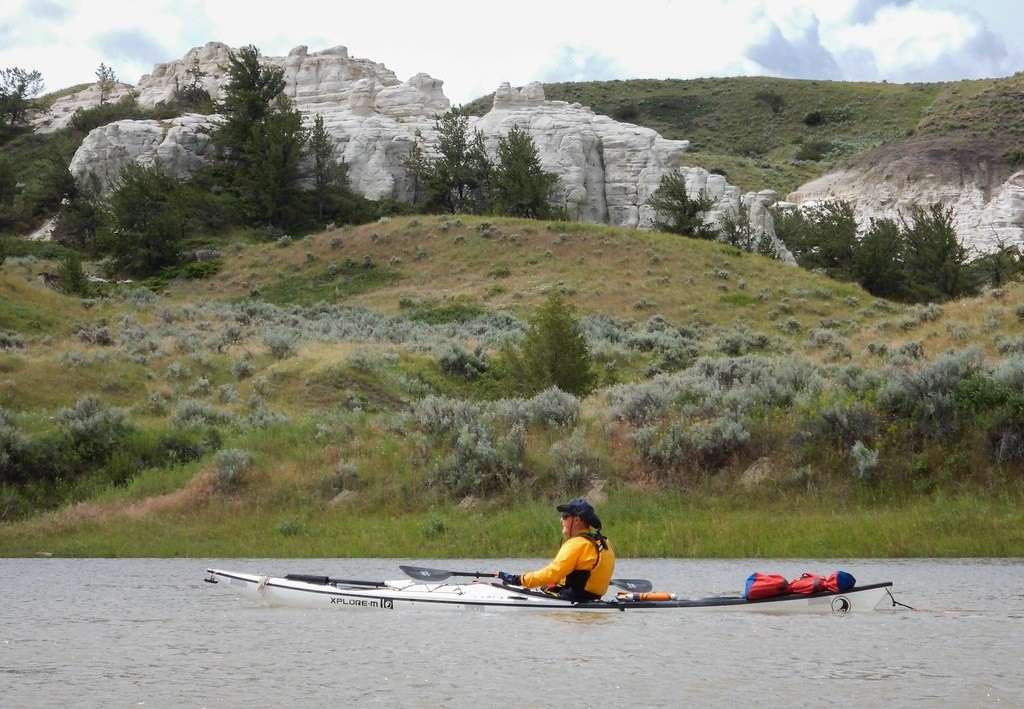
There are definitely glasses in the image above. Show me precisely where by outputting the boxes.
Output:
[562,512,574,520]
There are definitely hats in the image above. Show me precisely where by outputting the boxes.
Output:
[557,498,602,529]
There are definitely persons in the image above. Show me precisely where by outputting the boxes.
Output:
[492,499,615,600]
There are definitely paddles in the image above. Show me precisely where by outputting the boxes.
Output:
[398,566,653,596]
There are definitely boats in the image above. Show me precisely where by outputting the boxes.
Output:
[207,498,893,614]
